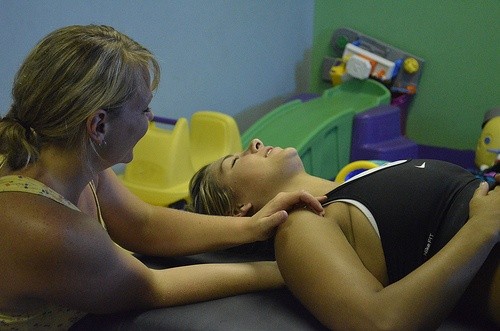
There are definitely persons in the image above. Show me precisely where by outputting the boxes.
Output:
[186,138,500,330]
[0,23,328,331]
